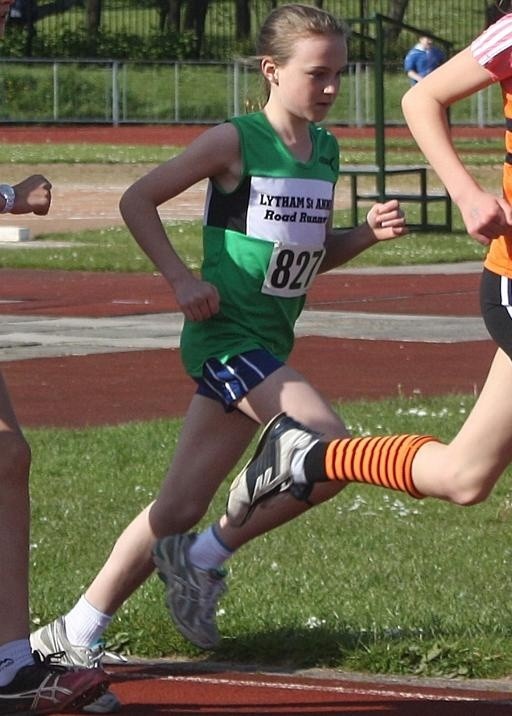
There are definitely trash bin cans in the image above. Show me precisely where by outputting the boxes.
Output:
[1,179,15,213]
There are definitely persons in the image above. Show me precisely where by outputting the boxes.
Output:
[31,4,406,715]
[401,24,447,91]
[223,10,512,533]
[1,172,109,715]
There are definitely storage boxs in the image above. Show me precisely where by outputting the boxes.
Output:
[336,160,454,234]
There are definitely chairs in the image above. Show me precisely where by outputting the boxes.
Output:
[226,412,313,528]
[0,613,120,716]
[152,532,228,650]
